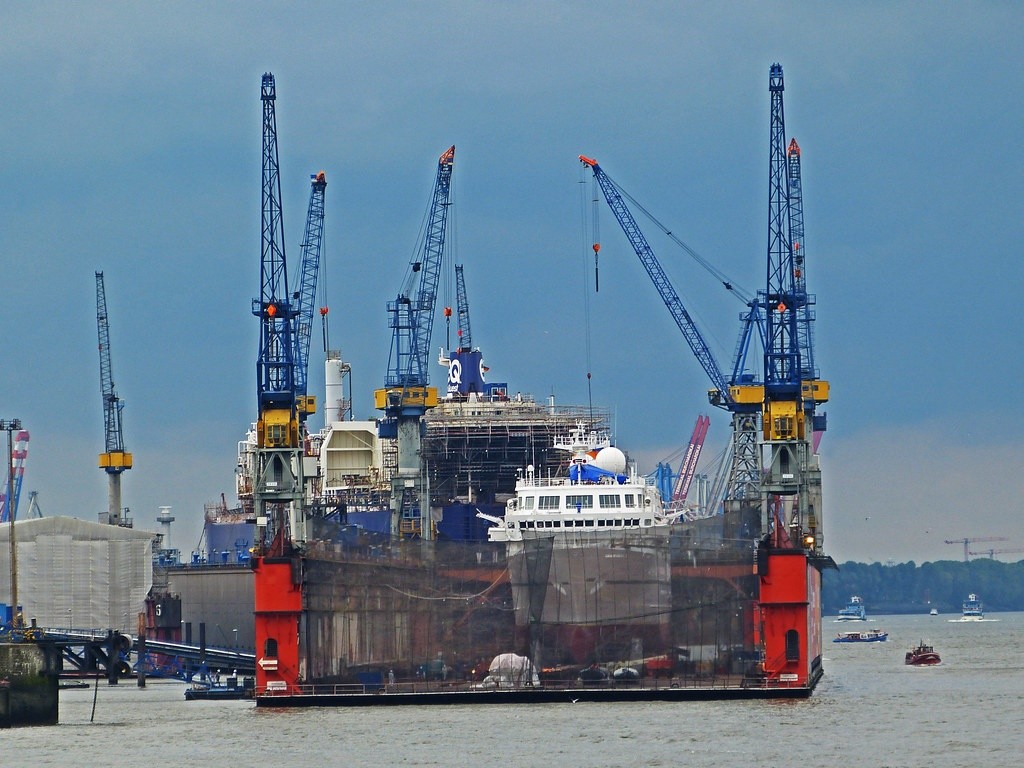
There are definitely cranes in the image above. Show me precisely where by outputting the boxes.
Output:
[445,262,514,401]
[767,63,828,548]
[579,152,768,546]
[374,146,456,440]
[251,72,334,554]
[944,536,1010,561]
[969,549,1023,560]
[91,269,134,526]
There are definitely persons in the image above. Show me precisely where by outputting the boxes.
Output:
[232,670,237,678]
[388,670,394,684]
[419,666,424,678]
[576,501,581,512]
[463,664,481,681]
[214,669,220,683]
[442,664,447,680]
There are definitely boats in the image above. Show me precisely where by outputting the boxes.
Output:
[832,628,888,643]
[930,608,938,615]
[905,638,941,666]
[475,420,673,665]
[184,678,256,701]
[961,593,984,621]
[837,596,867,621]
